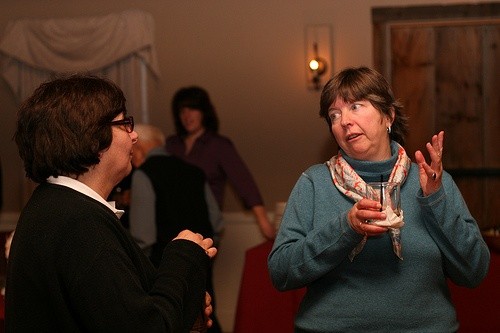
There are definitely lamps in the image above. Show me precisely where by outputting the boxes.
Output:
[309,55,327,75]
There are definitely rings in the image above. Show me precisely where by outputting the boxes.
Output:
[358,222,362,230]
[432,173,436,181]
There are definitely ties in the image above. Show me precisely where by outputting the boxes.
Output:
[324,138,411,262]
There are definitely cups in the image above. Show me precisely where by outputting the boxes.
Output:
[366,182,402,225]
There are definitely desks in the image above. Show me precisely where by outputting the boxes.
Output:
[232,237,500,333]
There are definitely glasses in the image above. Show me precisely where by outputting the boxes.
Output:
[111,116,134,133]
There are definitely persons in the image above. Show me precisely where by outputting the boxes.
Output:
[118,86,278,289]
[268,67,491,333]
[6,74,218,333]
